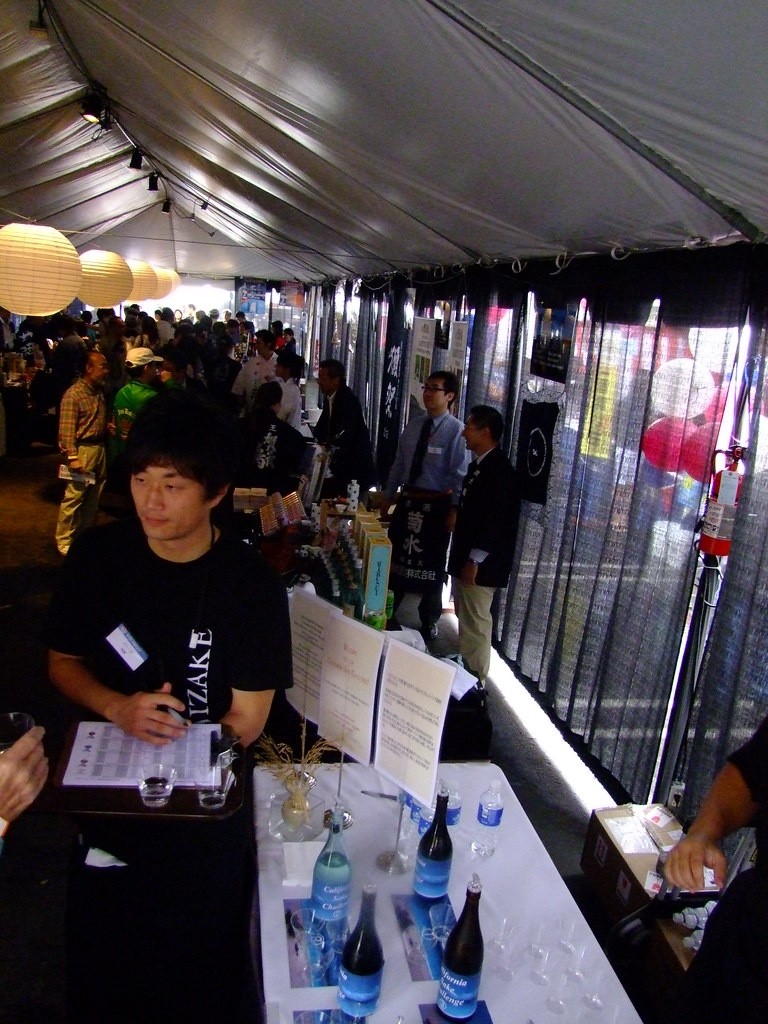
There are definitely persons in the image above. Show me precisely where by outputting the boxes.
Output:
[0,303,641,1024]
[660,712,768,1023]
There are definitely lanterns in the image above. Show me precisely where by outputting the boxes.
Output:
[0,222,182,317]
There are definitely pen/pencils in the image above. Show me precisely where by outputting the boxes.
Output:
[144,689,188,727]
[361,791,397,800]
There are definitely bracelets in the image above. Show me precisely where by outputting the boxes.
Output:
[0,817,8,835]
[68,456,77,459]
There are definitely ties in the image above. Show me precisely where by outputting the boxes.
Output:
[408,417,432,485]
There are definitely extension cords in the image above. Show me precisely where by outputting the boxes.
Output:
[668,781,685,807]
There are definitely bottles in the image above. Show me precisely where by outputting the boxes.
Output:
[298,574,316,595]
[436,873,484,1022]
[673,899,720,950]
[309,794,351,921]
[472,779,504,856]
[311,503,365,621]
[413,778,453,903]
[396,775,462,851]
[335,883,386,1017]
[8,351,25,373]
[348,479,360,512]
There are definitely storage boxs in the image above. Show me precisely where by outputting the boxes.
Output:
[575,804,717,932]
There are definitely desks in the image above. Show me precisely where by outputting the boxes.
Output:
[253,762,642,1024]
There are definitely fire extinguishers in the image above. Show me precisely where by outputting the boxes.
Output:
[693,436,748,555]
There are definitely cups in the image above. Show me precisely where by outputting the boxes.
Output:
[403,904,605,1014]
[0,712,36,750]
[133,763,178,807]
[192,766,235,808]
[335,504,345,512]
[291,907,356,1023]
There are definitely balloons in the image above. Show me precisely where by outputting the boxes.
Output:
[643,320,753,481]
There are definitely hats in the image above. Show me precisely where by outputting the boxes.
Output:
[125,348,164,368]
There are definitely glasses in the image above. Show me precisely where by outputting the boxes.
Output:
[421,386,446,392]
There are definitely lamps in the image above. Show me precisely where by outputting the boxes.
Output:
[147,175,160,191]
[201,195,212,210]
[161,199,171,214]
[80,90,118,142]
[127,147,144,170]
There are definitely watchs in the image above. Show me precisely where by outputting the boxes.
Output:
[469,558,479,566]
